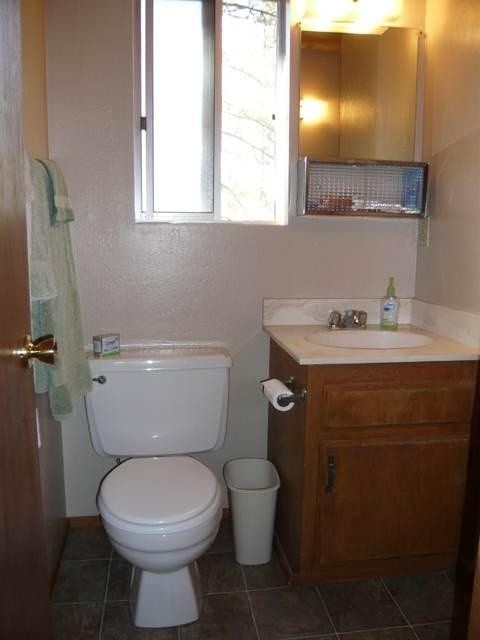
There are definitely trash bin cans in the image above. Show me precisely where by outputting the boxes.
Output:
[223,457,280,566]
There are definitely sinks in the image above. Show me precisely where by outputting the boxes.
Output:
[305,328,432,349]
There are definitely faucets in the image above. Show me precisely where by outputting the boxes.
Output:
[342,309,360,326]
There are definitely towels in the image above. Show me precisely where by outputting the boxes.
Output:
[25,155,94,421]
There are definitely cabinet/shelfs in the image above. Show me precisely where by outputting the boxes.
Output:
[266,337,478,586]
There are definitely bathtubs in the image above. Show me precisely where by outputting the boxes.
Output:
[81,343,234,630]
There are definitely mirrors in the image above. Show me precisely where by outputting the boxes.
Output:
[298,20,421,162]
